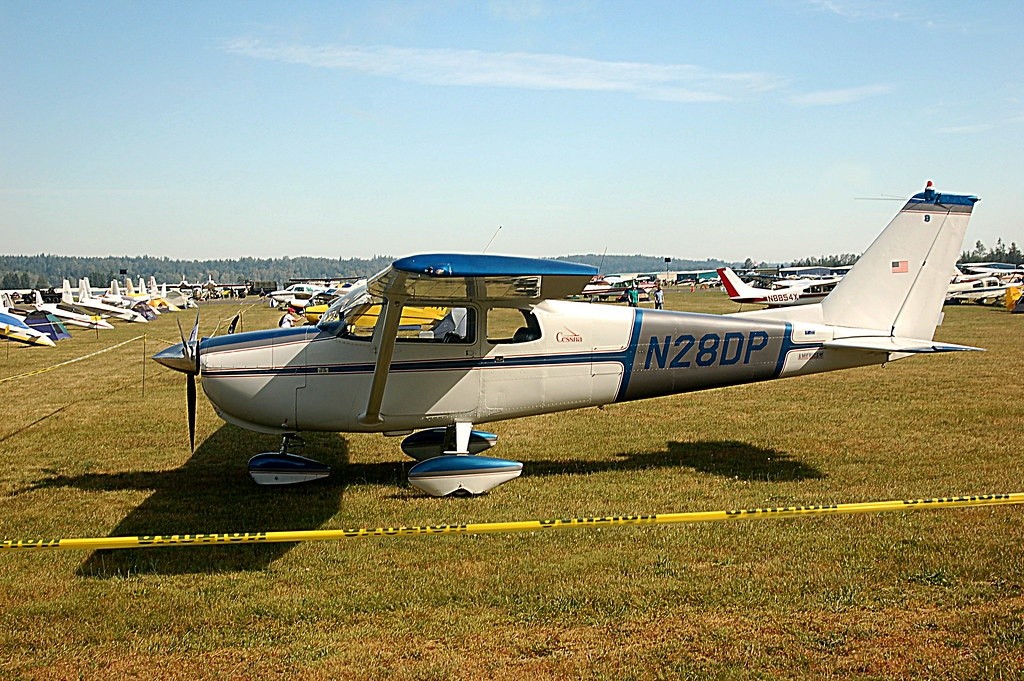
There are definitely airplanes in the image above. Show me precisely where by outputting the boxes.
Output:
[513,277,658,301]
[716,266,1024,306]
[150,182,988,497]
[267,281,449,331]
[0,275,199,348]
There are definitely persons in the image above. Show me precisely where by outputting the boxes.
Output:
[989,272,994,277]
[1014,276,1024,285]
[628,286,638,307]
[653,286,664,310]
[140,302,147,317]
[260,286,264,294]
[278,308,304,328]
[691,282,694,293]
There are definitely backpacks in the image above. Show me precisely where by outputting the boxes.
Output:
[279,313,289,327]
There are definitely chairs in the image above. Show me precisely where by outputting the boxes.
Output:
[442,333,461,344]
[513,327,540,343]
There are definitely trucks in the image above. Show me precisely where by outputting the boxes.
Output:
[22,287,62,304]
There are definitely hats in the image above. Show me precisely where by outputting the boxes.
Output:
[288,308,296,313]
